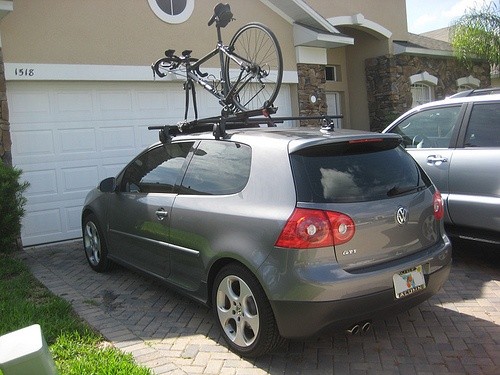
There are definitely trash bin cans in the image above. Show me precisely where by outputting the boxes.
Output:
[0,323,57,375]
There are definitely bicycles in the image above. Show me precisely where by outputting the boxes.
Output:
[149,3,285,117]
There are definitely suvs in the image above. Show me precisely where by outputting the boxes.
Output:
[379,87,500,245]
[78,114,455,359]
